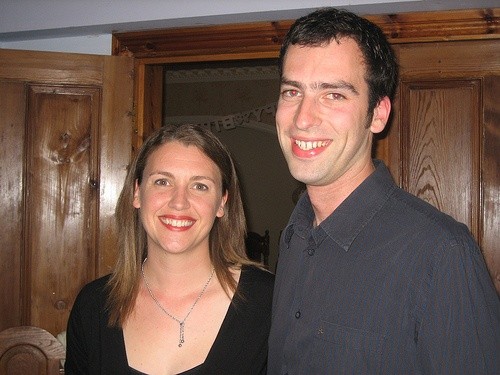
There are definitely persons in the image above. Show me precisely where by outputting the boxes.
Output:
[63,121,274,375]
[268,8,499,375]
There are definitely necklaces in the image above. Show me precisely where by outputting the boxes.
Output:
[141,257,217,347]
